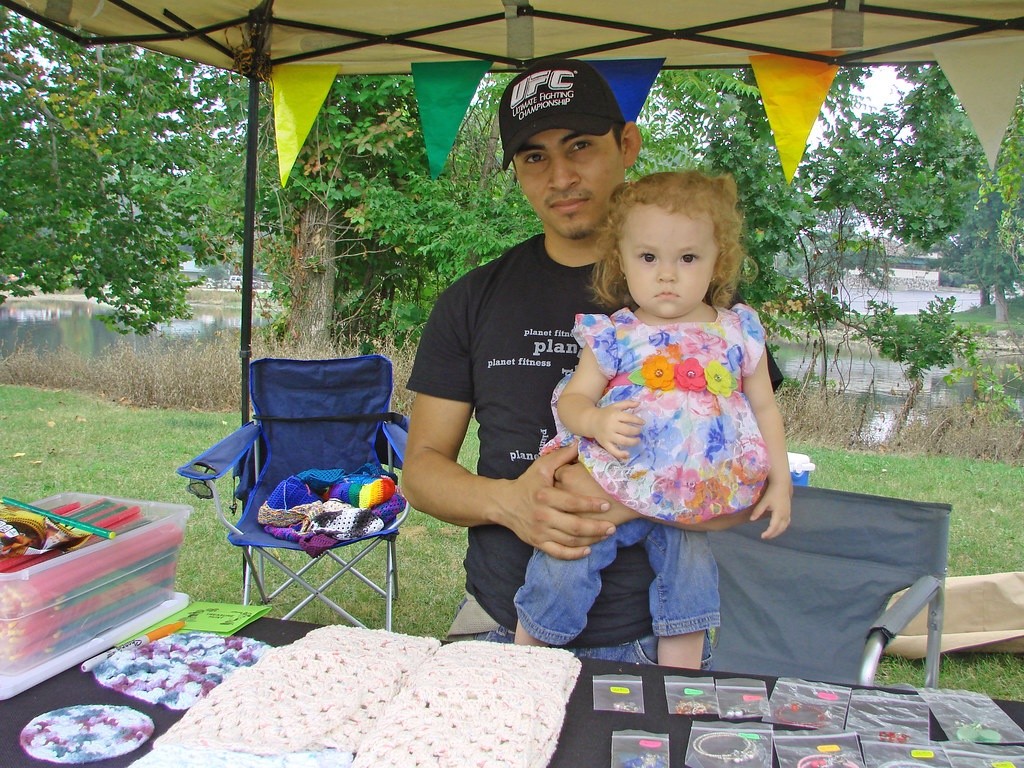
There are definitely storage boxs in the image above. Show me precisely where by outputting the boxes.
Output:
[0,490,192,699]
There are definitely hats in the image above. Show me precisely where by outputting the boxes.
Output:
[499,58,625,172]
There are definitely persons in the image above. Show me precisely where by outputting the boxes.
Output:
[515,171,793,676]
[400,59,783,672]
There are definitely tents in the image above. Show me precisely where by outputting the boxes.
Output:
[0,0,1023,589]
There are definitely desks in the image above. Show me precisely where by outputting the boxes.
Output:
[0,602,1024,768]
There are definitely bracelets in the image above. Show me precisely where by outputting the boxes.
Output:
[692,700,939,768]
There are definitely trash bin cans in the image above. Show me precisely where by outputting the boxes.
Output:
[786,451,816,485]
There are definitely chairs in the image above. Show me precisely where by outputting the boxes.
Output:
[177,355,414,634]
[671,480,953,690]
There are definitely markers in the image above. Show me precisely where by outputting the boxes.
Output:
[81,620,186,673]
